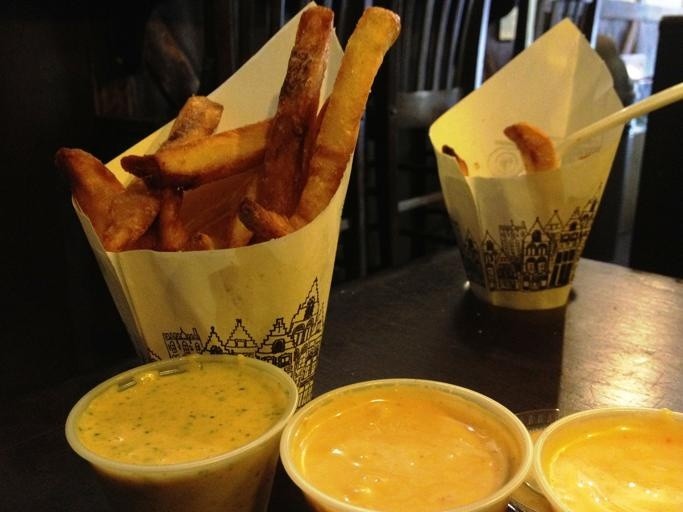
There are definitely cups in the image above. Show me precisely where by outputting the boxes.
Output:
[277,380,535,512]
[503,405,580,511]
[62,352,301,512]
[531,406,683,512]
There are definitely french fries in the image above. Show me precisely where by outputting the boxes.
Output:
[53,6,402,253]
[441,122,557,177]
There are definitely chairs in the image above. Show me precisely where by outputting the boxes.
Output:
[226,0,492,293]
[511,0,599,59]
